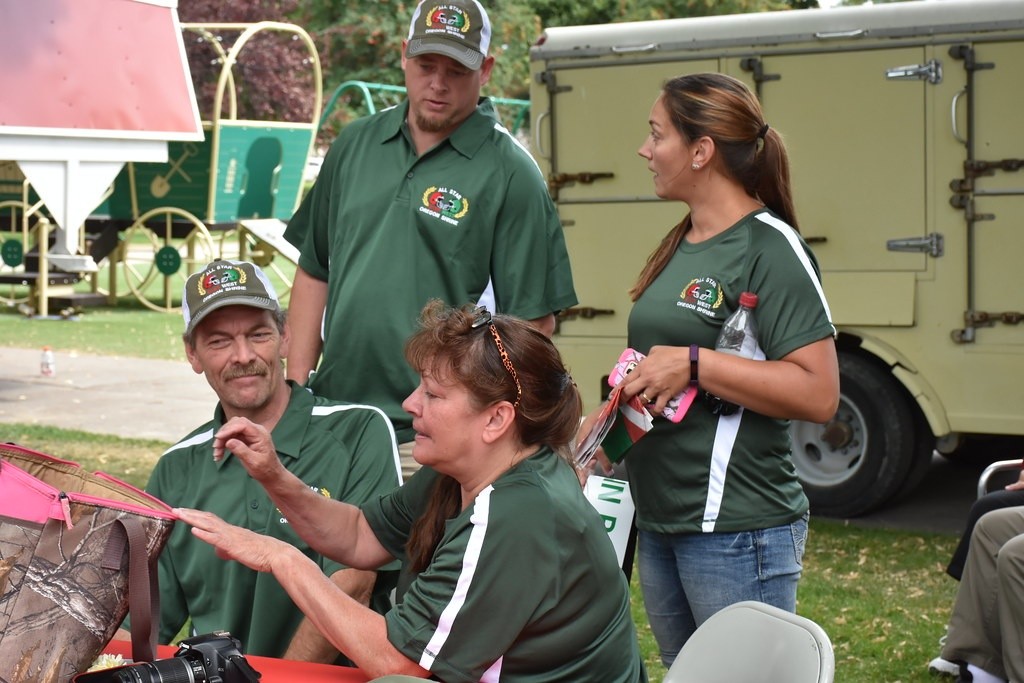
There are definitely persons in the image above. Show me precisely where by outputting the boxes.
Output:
[172,300,649,683]
[284,0,579,615]
[941,505,1024,683]
[109,259,404,668]
[928,467,1024,677]
[573,73,839,670]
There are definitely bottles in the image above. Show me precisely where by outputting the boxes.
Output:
[702,291,760,416]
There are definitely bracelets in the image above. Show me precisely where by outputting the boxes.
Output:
[689,344,699,384]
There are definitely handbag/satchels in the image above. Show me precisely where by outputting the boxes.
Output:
[0,440,179,683]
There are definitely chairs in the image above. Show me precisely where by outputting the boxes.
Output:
[660,600,835,683]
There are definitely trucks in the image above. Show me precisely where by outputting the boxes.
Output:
[528,0,1024,520]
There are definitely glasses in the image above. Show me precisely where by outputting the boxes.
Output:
[468,304,523,410]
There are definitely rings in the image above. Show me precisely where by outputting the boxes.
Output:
[642,392,651,402]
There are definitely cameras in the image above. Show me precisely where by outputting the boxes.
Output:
[76,629,248,683]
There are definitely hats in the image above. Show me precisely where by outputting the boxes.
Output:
[405,0,492,70]
[179,257,280,336]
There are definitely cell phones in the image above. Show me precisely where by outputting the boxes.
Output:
[608,347,699,423]
